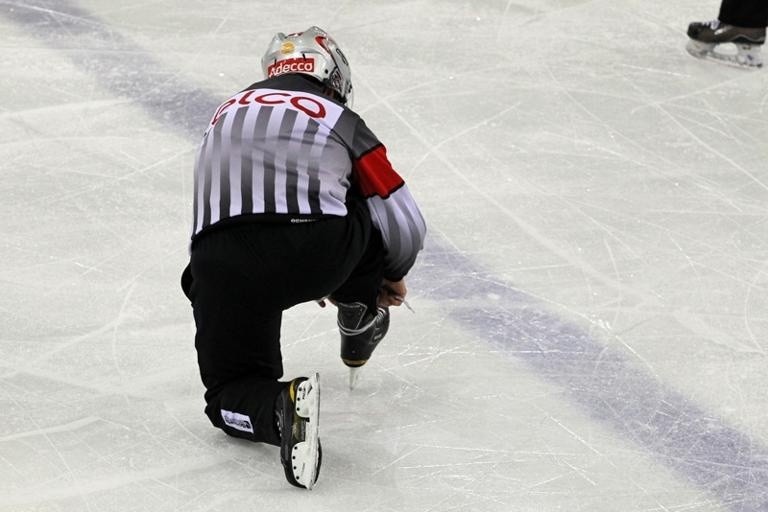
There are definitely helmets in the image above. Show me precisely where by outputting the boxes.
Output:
[260,26,353,109]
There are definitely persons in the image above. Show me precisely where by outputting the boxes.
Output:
[178,26,428,490]
[686,0,768,47]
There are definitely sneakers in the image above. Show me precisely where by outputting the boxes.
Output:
[275,377,321,488]
[686,18,765,44]
[336,295,415,368]
